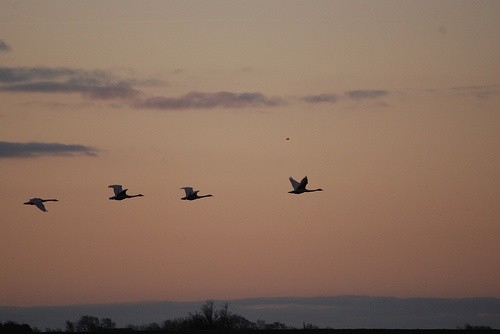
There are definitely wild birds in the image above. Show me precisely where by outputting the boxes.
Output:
[287,176,324,194]
[179,186,213,200]
[107,184,144,201]
[23,197,59,212]
